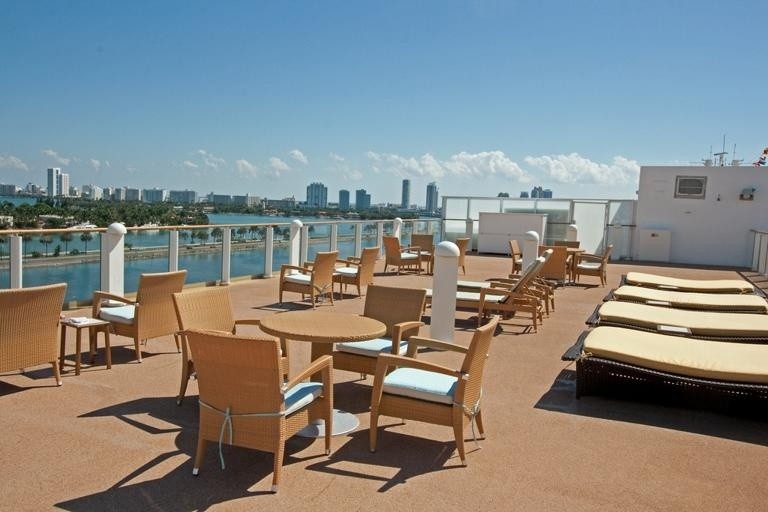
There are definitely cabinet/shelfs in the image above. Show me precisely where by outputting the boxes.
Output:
[476,212,549,259]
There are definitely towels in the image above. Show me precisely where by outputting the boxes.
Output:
[68,316,88,324]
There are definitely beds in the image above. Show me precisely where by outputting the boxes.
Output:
[603,284,768,315]
[585,301,768,342]
[619,272,766,299]
[561,326,768,400]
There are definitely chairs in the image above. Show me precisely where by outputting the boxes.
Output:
[407,234,433,275]
[0,282,68,386]
[555,240,580,248]
[333,285,426,377]
[172,284,290,405]
[573,244,615,288]
[185,327,333,494]
[369,314,501,467]
[431,238,470,275]
[423,256,546,333]
[89,268,188,363]
[279,251,339,310]
[538,245,567,288]
[509,239,523,275]
[457,248,555,318]
[382,236,421,276]
[335,247,380,300]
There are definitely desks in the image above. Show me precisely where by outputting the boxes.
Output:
[566,247,585,284]
[60,316,112,376]
[330,273,343,300]
[260,310,387,439]
